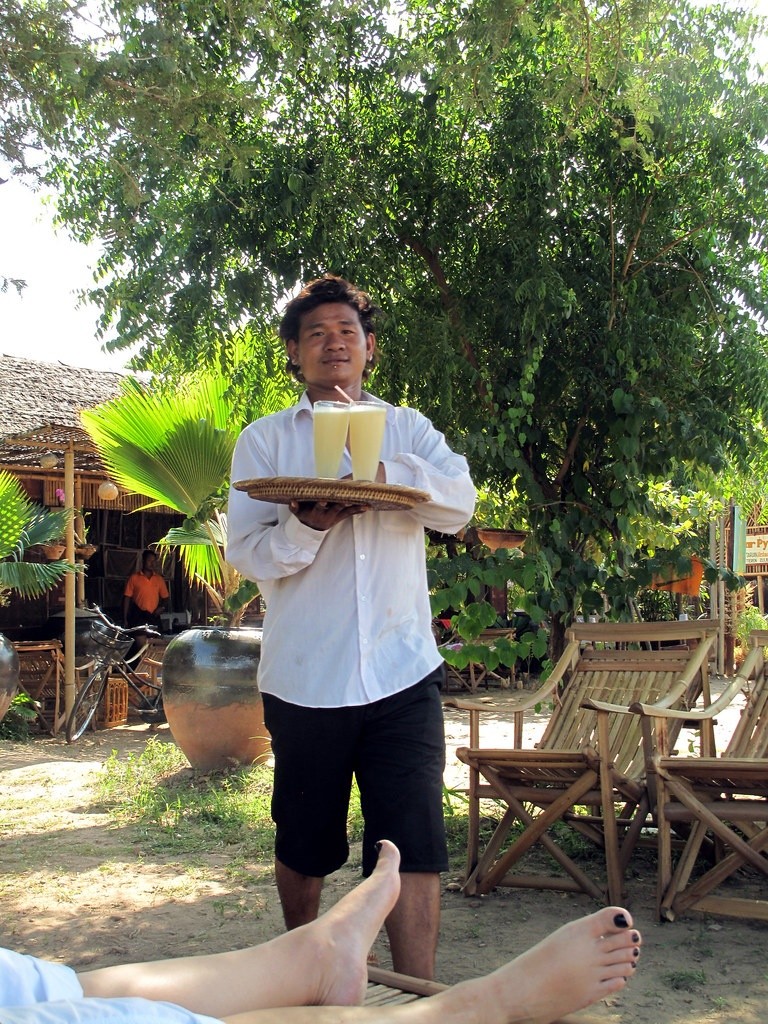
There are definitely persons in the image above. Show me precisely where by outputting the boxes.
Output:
[125,549,170,634]
[226,278,478,982]
[0,840,643,1024]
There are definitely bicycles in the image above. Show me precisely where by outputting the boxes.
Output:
[64,602,162,744]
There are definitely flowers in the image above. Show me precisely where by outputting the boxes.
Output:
[54,488,91,548]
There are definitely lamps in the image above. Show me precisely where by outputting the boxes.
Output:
[40,431,58,468]
[97,472,119,502]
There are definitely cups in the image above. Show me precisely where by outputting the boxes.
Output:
[346,401,388,483]
[314,400,351,479]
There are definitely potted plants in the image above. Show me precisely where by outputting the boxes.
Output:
[82,373,271,771]
[477,503,532,550]
[651,533,709,596]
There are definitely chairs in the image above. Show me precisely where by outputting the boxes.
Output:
[628,630,768,929]
[442,619,724,907]
[13,637,172,735]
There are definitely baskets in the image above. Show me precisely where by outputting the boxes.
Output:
[85,621,134,665]
